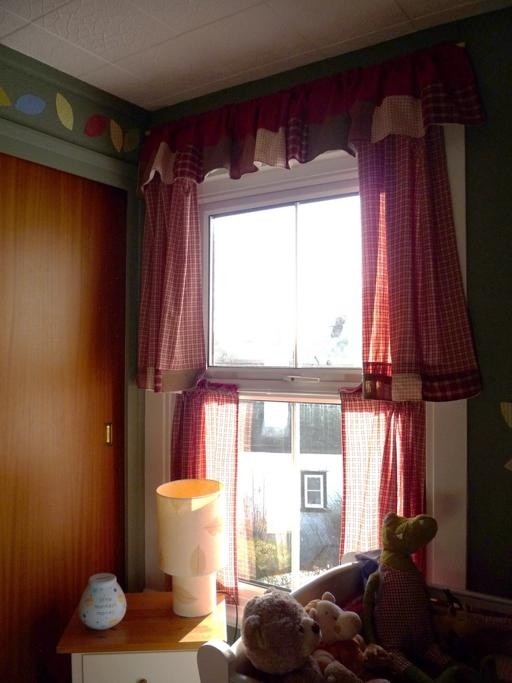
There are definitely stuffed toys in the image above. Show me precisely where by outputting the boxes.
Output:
[304,591,391,682]
[351,511,497,683]
[241,586,364,683]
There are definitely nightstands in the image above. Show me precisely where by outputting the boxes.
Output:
[56,590,227,683]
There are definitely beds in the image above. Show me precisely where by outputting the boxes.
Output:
[195,549,512,682]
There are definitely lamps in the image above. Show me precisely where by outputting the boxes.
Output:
[155,478,223,619]
